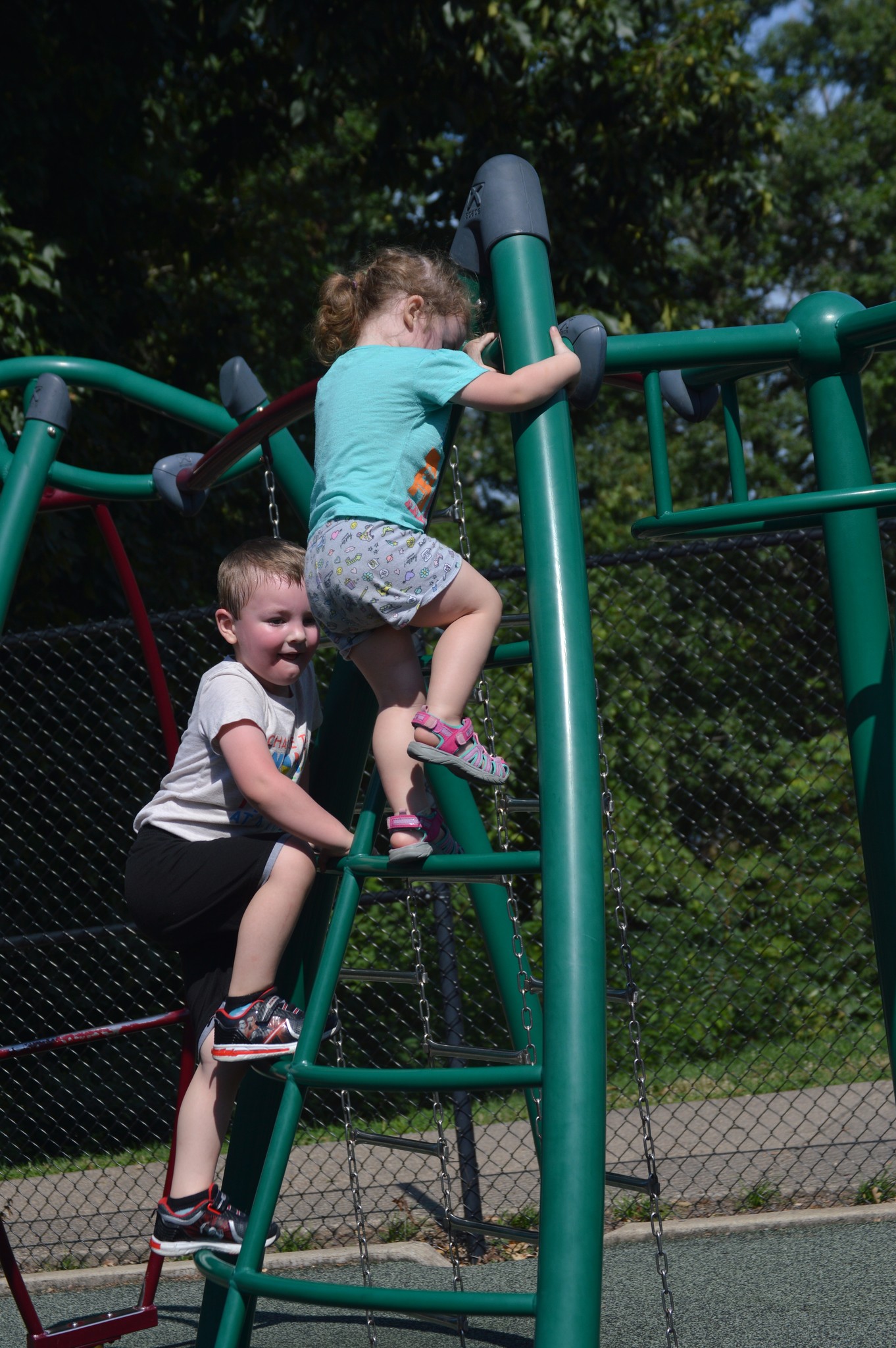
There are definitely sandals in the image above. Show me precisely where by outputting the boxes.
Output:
[408,708,510,784]
[386,813,472,867]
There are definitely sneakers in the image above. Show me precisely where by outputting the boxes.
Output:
[210,992,347,1063]
[148,1183,283,1258]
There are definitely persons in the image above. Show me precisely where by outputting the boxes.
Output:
[124,538,378,1256]
[303,244,582,864]
